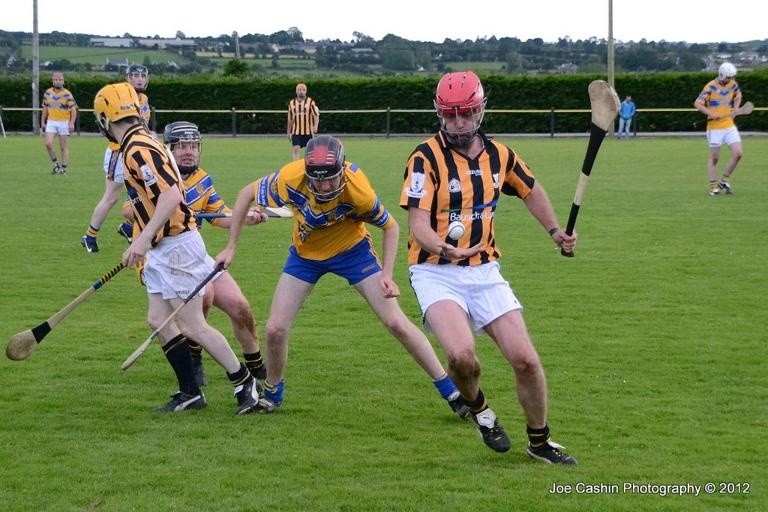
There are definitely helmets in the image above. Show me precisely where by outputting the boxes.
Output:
[719,63,738,84]
[124,64,149,93]
[162,120,202,174]
[93,82,141,133]
[303,134,347,204]
[432,70,489,148]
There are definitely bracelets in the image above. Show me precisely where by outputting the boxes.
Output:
[549,228,560,236]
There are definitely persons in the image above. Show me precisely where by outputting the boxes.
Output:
[81,63,150,254]
[91,82,263,416]
[399,70,577,469]
[617,96,636,140]
[41,73,79,176]
[286,83,319,161]
[213,134,472,419]
[694,62,742,196]
[121,120,268,386]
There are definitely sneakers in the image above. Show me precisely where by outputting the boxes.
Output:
[709,181,719,197]
[191,362,209,387]
[718,179,733,194]
[117,222,134,244]
[50,166,60,174]
[469,404,512,454]
[149,389,207,416]
[253,394,283,415]
[446,392,472,419]
[79,235,100,255]
[245,364,267,380]
[233,374,259,415]
[526,441,578,467]
[60,169,67,176]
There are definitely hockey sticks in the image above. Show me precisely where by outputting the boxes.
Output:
[6,262,123,360]
